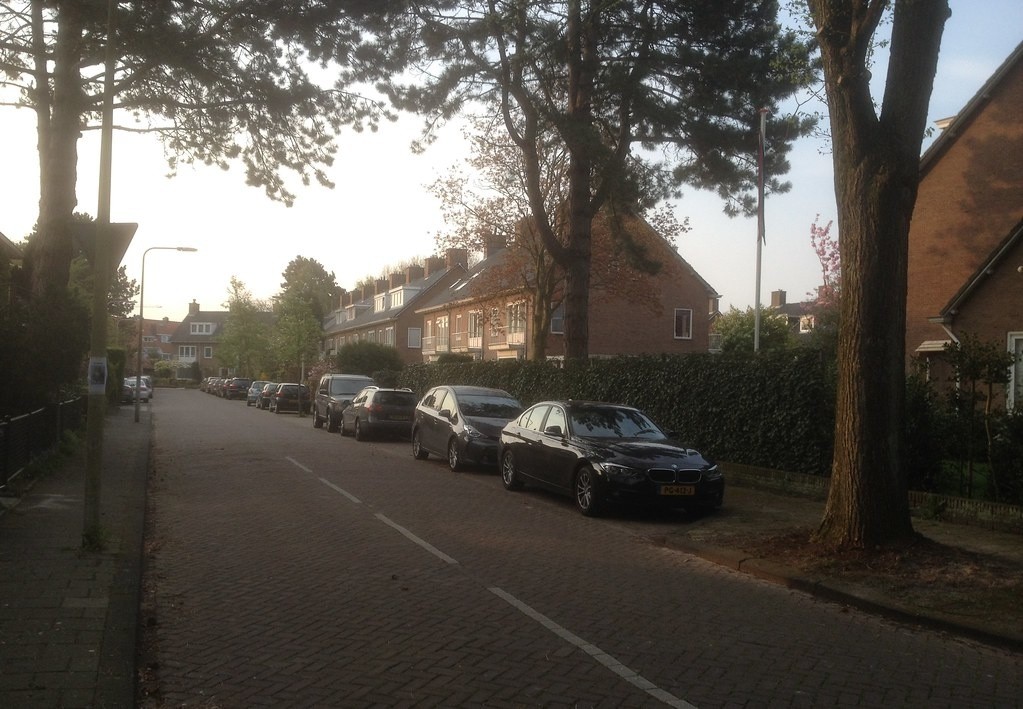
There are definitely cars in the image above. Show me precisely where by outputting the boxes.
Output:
[404,381,530,474]
[204,365,313,414]
[338,385,420,441]
[120,370,154,404]
[495,398,723,526]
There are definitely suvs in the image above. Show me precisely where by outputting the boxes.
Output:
[307,375,379,425]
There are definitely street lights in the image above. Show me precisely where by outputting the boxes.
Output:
[134,246,203,418]
